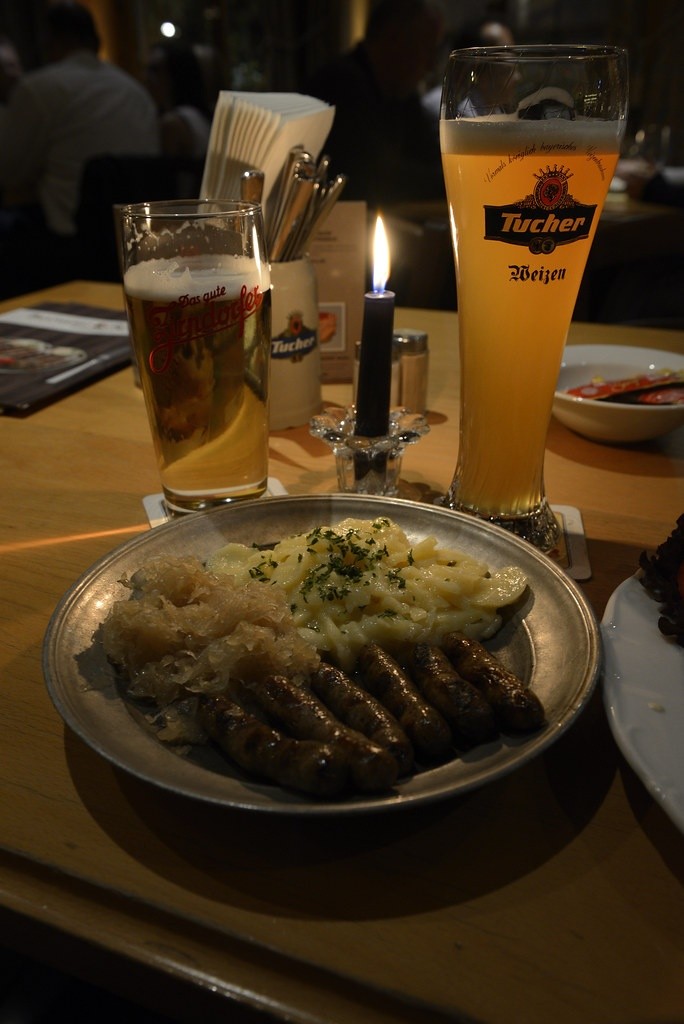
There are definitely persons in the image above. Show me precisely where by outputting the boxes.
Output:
[1,0,684,293]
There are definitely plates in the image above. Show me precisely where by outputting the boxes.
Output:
[600,552,683,832]
[43,492,603,815]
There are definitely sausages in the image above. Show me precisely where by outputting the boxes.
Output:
[196,631,545,798]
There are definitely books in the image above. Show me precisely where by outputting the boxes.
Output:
[0,302,135,416]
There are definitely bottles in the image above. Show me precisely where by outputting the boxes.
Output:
[352,329,427,417]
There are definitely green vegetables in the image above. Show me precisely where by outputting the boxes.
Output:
[249,519,416,620]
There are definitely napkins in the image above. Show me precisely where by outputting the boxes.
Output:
[196,90,335,235]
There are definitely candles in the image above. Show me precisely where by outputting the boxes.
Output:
[355,215,395,481]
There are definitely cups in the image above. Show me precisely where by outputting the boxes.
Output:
[631,125,667,192]
[115,198,275,518]
[263,256,324,435]
[437,41,629,558]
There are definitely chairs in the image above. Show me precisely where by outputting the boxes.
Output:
[71,153,190,281]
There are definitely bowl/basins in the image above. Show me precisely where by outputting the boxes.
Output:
[550,344,683,443]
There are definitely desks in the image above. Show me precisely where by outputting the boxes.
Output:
[0,279,684,1024]
[392,192,683,278]
